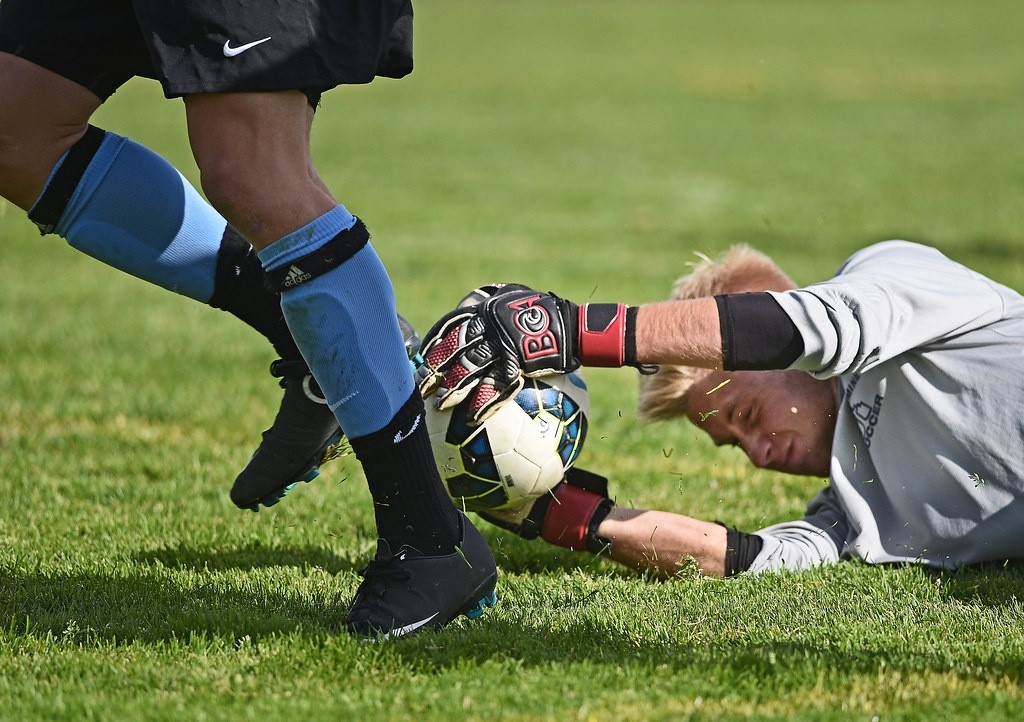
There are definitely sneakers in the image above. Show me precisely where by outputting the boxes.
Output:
[229,359,347,512]
[327,508,498,645]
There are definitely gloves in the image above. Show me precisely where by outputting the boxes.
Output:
[471,464,615,557]
[410,282,627,426]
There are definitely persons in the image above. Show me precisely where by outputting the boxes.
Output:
[409,240,1023,584]
[0,0,501,638]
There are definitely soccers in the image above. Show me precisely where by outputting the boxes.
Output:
[422,367,589,511]
